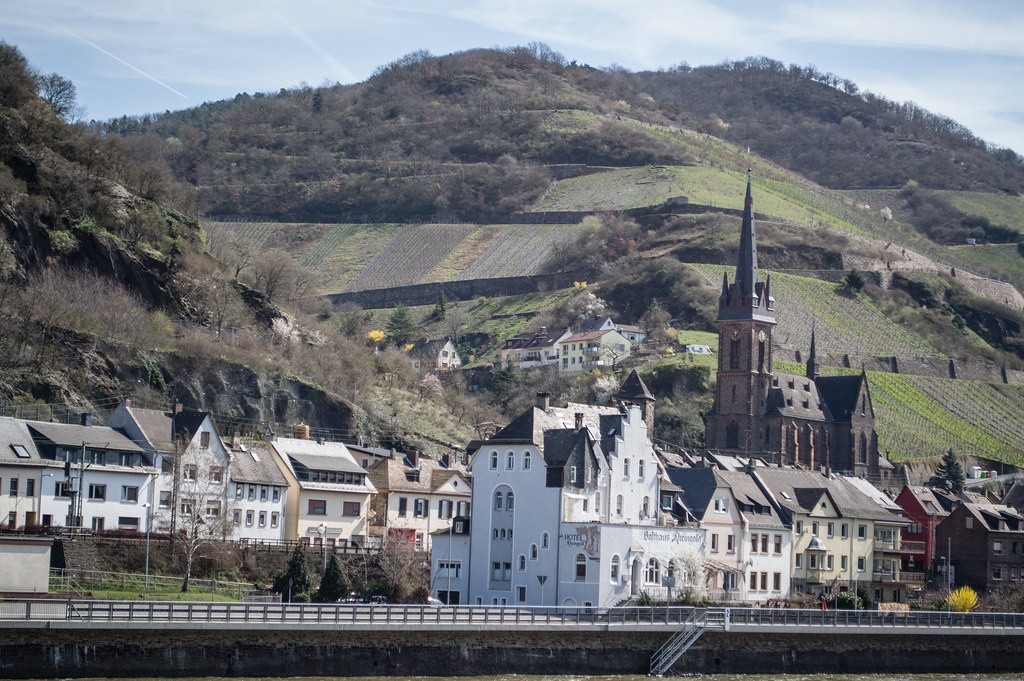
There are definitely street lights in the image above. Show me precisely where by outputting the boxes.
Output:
[446,520,452,606]
[317,521,329,576]
[144,502,152,589]
[38,474,54,527]
